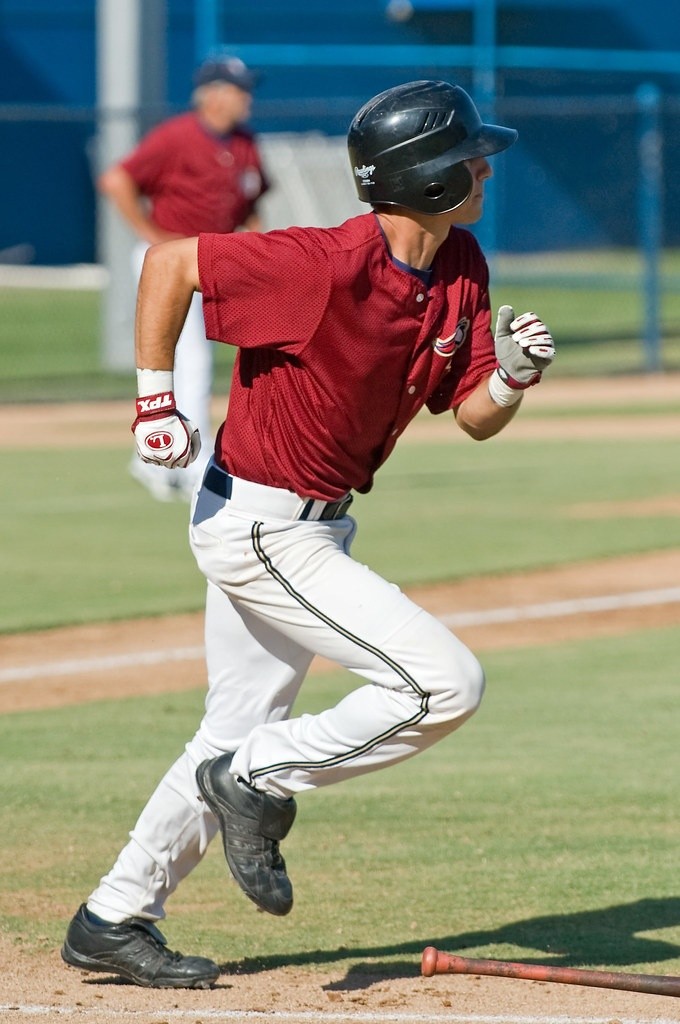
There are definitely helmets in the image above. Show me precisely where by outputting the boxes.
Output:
[349,80,518,214]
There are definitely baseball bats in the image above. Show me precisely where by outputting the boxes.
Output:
[420,945,680,998]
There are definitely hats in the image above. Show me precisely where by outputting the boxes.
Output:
[194,61,264,93]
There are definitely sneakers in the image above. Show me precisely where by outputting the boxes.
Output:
[62,904,220,987]
[196,752,296,915]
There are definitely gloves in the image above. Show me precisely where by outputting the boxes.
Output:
[486,303,557,407]
[130,369,202,470]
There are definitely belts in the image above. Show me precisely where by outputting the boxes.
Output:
[204,467,355,520]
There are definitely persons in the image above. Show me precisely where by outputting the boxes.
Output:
[95,57,271,506]
[60,80,554,990]
